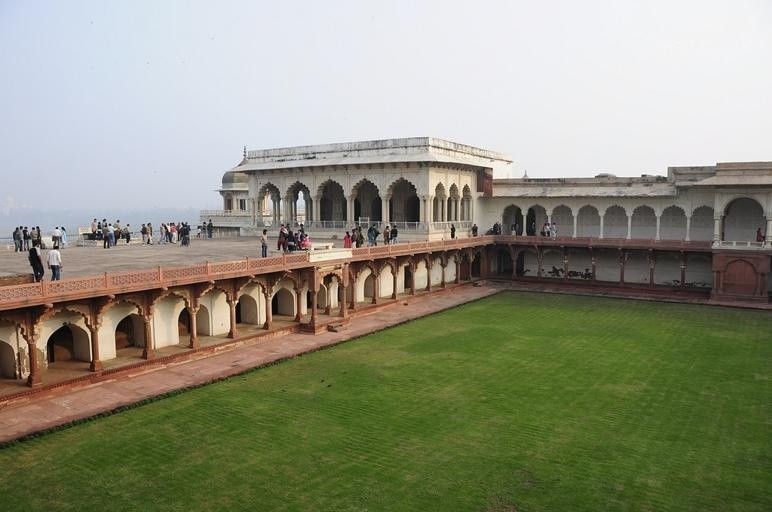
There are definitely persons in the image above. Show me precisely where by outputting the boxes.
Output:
[757,228,763,242]
[543,222,560,236]
[277,222,309,253]
[471,224,478,236]
[48,246,64,282]
[91,216,215,249]
[485,221,503,234]
[61,227,67,249]
[12,225,45,253]
[53,227,63,249]
[529,221,536,235]
[260,229,269,257]
[514,222,519,234]
[451,224,456,238]
[28,243,46,282]
[343,223,398,249]
